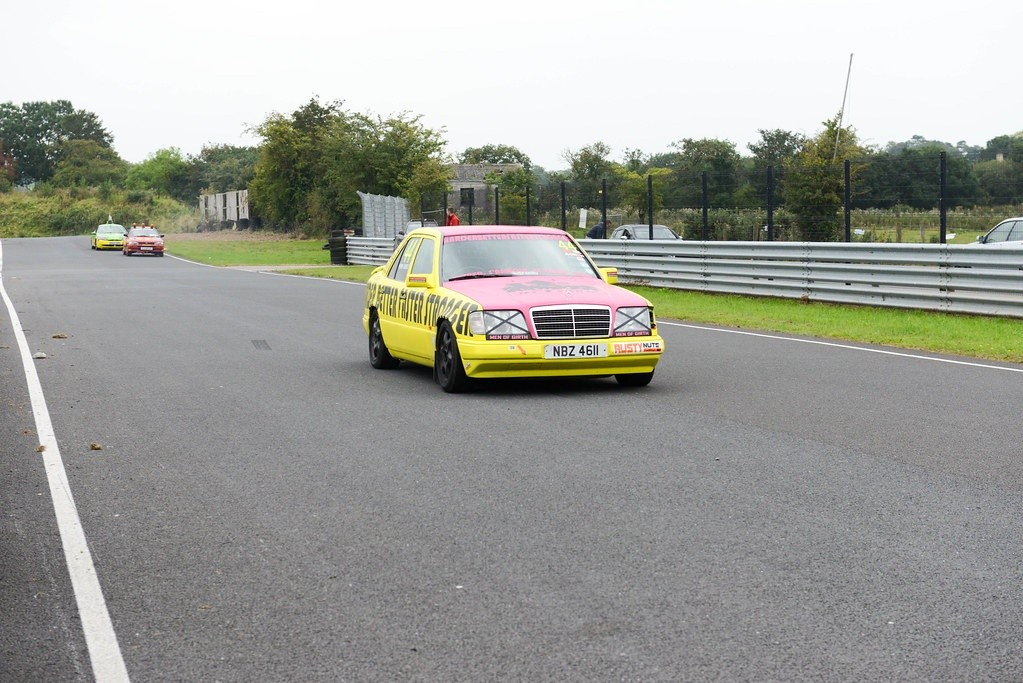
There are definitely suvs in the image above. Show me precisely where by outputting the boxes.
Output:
[397,218,441,236]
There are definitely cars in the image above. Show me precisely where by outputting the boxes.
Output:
[122,222,165,257]
[363,223,664,397]
[90,224,127,251]
[609,223,687,240]
[958,216,1023,247]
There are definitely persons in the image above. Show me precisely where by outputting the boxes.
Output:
[445,207,459,226]
[584,219,612,239]
